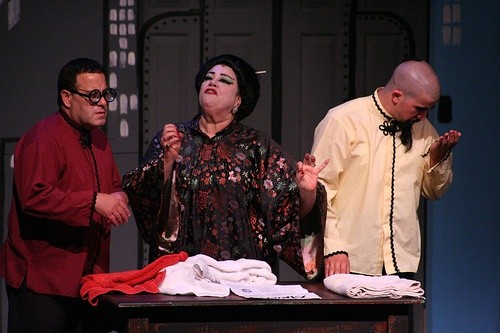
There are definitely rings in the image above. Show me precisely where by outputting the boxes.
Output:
[311,164,316,167]
[165,143,170,150]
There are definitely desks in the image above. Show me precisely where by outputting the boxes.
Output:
[97,279,427,333]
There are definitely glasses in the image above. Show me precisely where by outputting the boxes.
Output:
[72,88,117,104]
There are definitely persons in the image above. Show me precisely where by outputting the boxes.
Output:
[309,60,462,282]
[0,58,131,333]
[121,55,330,284]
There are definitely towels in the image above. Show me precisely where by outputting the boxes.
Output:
[158,254,230,298]
[192,258,277,285]
[79,251,188,306]
[323,274,426,299]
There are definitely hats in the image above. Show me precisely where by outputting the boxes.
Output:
[195,55,266,121]
